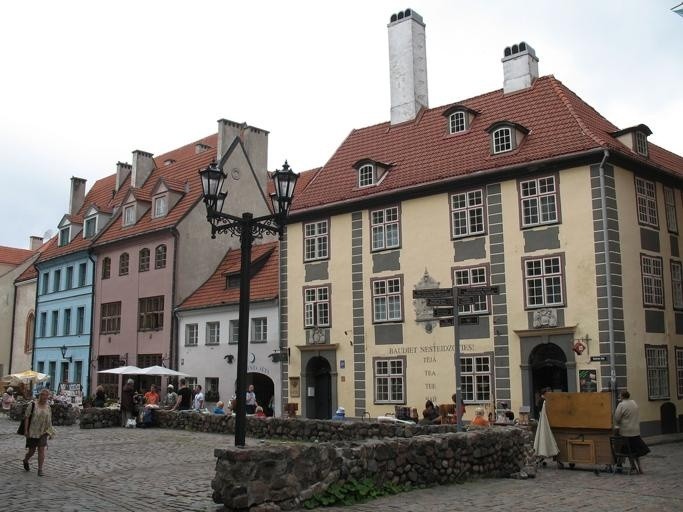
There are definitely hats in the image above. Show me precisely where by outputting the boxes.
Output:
[335,406,346,416]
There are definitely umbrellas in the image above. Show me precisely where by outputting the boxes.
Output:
[532,400,560,458]
[97,366,141,374]
[1,369,52,399]
[122,366,188,375]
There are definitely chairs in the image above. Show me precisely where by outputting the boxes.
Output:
[609,436,639,477]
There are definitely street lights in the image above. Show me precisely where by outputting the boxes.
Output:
[191,154,301,454]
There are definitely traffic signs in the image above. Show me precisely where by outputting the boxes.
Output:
[407,285,498,327]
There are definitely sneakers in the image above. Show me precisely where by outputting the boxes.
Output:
[23,459,30,471]
[37,468,44,476]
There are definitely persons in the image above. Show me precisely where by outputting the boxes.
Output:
[423,387,553,427]
[0,380,70,410]
[23,389,52,476]
[333,408,347,420]
[615,390,651,475]
[93,379,275,427]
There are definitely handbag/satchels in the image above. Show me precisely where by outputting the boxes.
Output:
[17,401,36,435]
